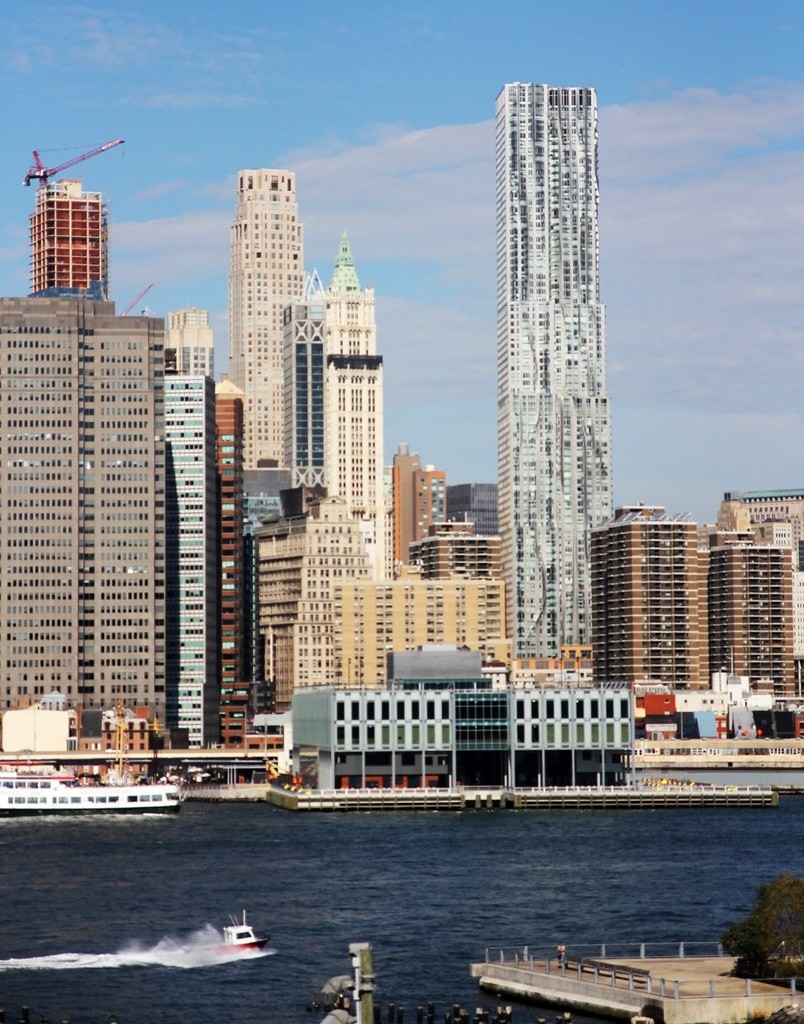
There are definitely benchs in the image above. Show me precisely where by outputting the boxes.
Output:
[568,956,650,978]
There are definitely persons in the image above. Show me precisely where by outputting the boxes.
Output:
[556,941,566,968]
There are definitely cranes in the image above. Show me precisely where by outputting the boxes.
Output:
[21,139,125,188]
[119,282,156,315]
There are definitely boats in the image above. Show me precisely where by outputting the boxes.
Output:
[181,909,270,957]
[0,764,183,819]
